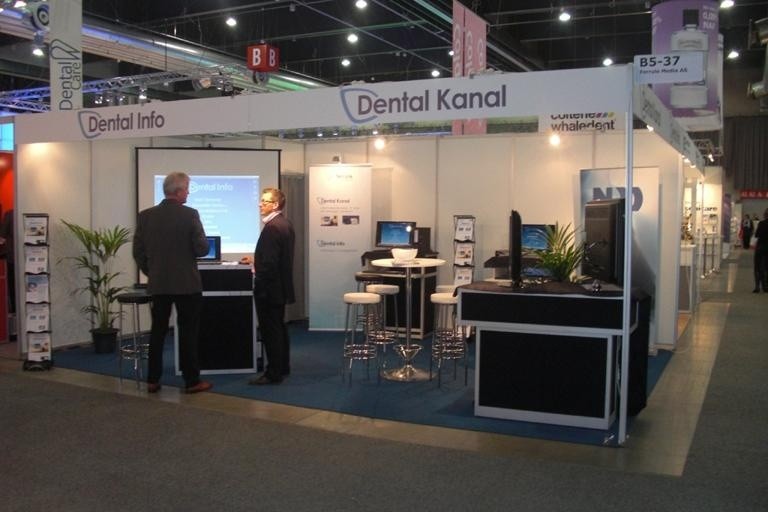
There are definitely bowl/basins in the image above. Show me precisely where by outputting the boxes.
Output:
[392,248,418,260]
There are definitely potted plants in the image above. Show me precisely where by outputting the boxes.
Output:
[52,216,137,355]
[525,219,602,295]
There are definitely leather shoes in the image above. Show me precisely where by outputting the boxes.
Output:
[763,288,768,292]
[752,288,760,293]
[266,367,292,375]
[250,373,279,386]
[148,382,162,395]
[184,384,212,395]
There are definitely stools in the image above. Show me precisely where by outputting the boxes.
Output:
[113,289,159,390]
[364,284,401,377]
[429,293,470,390]
[355,270,386,350]
[342,292,383,389]
[437,284,466,334]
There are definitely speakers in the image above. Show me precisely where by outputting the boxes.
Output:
[411,227,431,254]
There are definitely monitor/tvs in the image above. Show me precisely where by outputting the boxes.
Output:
[376,221,416,247]
[581,198,624,298]
[507,208,524,292]
[195,236,222,261]
[525,225,557,250]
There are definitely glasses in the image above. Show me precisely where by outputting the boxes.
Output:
[260,199,278,205]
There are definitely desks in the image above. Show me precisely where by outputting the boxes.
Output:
[371,258,447,383]
[456,279,638,432]
[492,249,555,282]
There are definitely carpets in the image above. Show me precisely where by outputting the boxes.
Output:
[0,251,768,512]
[44,313,676,447]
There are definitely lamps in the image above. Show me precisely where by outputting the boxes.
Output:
[93,91,103,105]
[226,14,237,27]
[138,85,148,100]
[341,58,351,68]
[347,33,360,44]
[719,0,736,11]
[224,77,234,93]
[431,69,440,77]
[355,0,368,10]
[726,50,740,64]
[215,75,224,91]
[557,7,571,23]
[448,50,455,56]
[31,31,46,56]
[602,57,613,66]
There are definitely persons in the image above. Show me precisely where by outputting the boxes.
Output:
[132,172,213,394]
[247,187,298,383]
[752,209,768,293]
[740,214,754,249]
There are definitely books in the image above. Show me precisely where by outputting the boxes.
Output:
[22,213,53,363]
[453,218,474,286]
